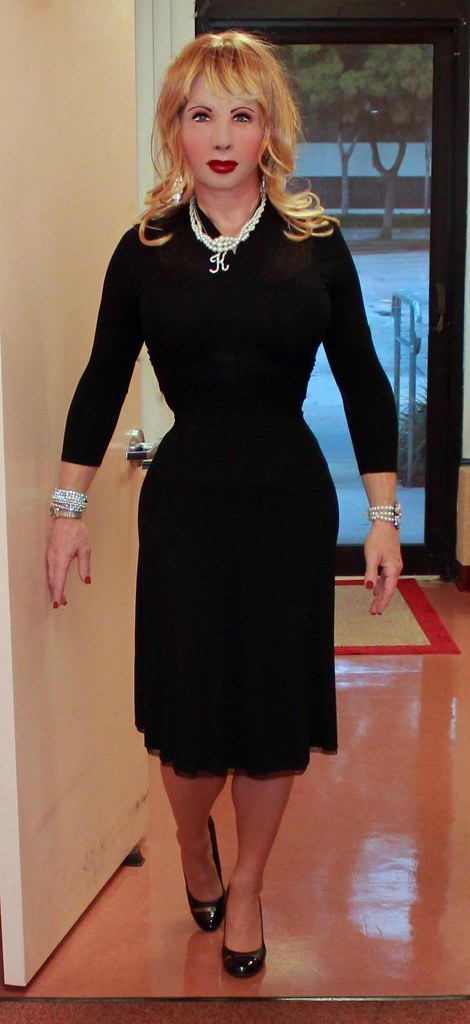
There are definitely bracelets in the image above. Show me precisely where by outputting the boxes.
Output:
[367,501,403,528]
[45,485,90,511]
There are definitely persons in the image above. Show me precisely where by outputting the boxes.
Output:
[44,33,402,974]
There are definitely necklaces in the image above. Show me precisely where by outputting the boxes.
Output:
[187,181,268,250]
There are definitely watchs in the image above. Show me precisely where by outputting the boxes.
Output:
[48,506,84,522]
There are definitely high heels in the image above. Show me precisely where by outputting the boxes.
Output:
[182,814,227,934]
[221,887,265,979]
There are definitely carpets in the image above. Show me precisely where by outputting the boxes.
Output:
[334,577,461,655]
